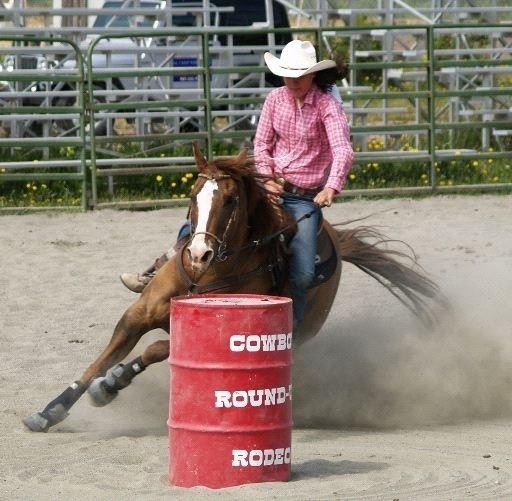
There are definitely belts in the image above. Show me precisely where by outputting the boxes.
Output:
[283,181,324,197]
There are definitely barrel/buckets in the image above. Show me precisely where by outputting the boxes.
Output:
[165,294,292,487]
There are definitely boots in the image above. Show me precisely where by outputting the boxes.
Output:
[120,272,154,293]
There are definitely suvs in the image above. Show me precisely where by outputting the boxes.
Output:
[0,0,294,137]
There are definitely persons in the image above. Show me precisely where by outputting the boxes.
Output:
[117,38,355,333]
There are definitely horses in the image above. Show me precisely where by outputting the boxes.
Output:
[21,141,452,435]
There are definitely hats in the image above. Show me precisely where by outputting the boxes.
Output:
[264,39,337,79]
